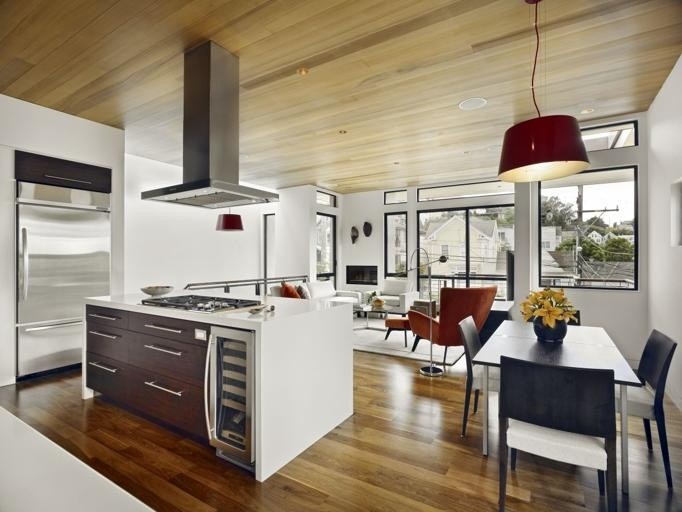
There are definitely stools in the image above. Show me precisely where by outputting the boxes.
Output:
[384,316,410,342]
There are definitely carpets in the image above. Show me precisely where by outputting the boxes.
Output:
[350,328,466,366]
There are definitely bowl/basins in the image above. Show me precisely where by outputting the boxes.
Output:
[140,285,174,297]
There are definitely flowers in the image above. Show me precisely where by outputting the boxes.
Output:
[516,285,581,328]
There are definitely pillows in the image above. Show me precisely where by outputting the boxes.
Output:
[279,281,337,300]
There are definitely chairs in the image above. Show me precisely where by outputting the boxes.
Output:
[406,282,498,380]
[364,278,420,315]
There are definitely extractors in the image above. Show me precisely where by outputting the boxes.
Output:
[140,39,280,210]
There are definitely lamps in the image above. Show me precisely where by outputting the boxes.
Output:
[216,205,246,233]
[496,0,590,184]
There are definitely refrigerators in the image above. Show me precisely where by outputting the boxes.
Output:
[15,148,113,379]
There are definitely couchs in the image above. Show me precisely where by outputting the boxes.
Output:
[268,278,362,316]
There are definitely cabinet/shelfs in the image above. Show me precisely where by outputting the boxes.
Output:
[129,311,210,444]
[83,303,130,417]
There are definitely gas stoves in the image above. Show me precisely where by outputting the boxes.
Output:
[142,292,260,314]
